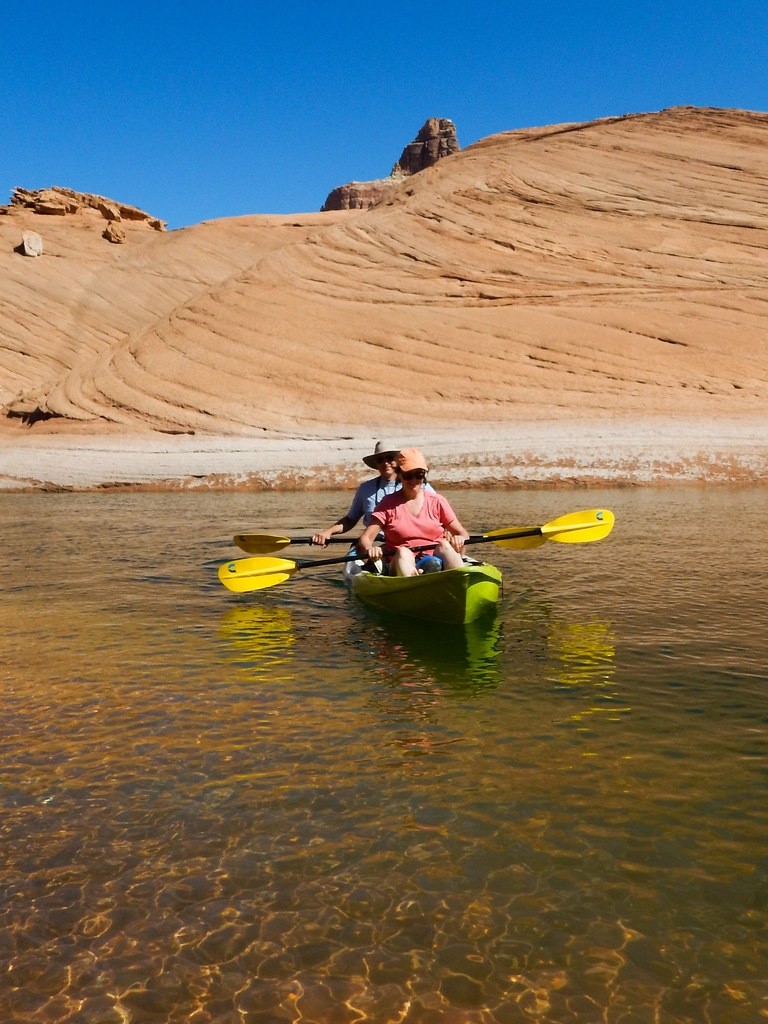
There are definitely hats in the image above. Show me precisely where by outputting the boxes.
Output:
[362,439,401,469]
[396,447,429,472]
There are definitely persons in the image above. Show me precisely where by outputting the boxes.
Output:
[312,439,452,549]
[356,447,469,577]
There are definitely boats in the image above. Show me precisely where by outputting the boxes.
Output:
[342,540,505,628]
[349,595,503,689]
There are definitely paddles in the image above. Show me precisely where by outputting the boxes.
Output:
[233,526,547,556]
[217,508,615,593]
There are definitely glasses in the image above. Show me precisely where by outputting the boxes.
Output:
[375,454,396,462]
[400,471,425,480]
